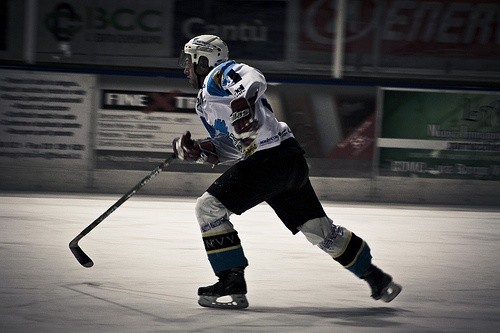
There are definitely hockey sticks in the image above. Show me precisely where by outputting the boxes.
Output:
[69,153,178,268]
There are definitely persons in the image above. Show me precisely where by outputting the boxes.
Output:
[172,34,402,308]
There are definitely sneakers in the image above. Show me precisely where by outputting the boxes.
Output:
[370,271,402,301]
[197,273,249,309]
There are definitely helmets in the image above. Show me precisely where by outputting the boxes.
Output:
[183,34,229,78]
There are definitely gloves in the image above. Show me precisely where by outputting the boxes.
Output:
[228,98,261,138]
[172,132,219,165]
